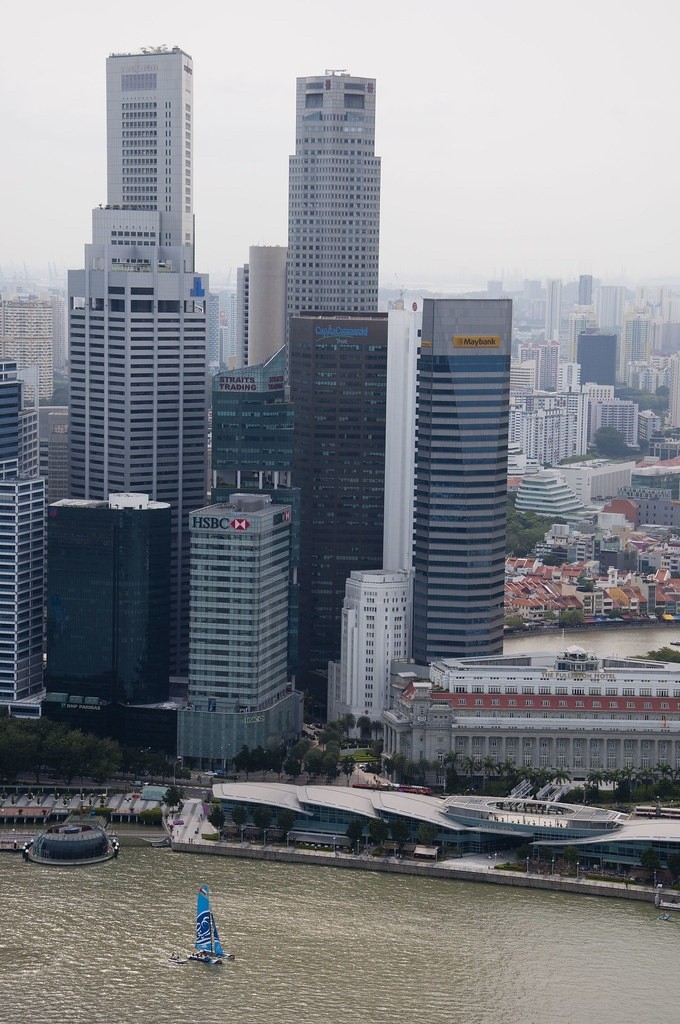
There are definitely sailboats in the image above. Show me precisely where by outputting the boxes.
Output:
[186,882,236,965]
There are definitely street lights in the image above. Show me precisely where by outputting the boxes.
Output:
[286,832,290,849]
[551,859,554,876]
[220,744,231,779]
[576,862,580,877]
[140,747,152,781]
[332,836,336,858]
[173,760,182,787]
[240,828,244,848]
[218,827,220,845]
[526,857,530,878]
[356,840,359,855]
[263,830,266,849]
[494,853,498,866]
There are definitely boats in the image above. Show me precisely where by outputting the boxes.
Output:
[168,957,188,965]
